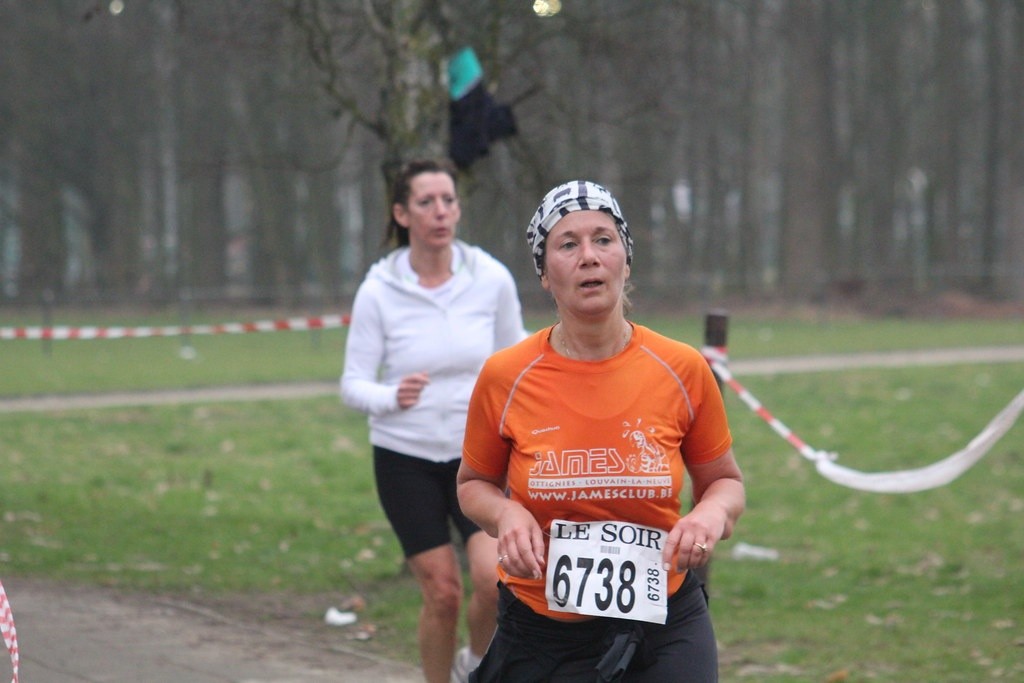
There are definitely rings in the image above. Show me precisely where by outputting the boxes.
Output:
[498,555,508,563]
[692,543,708,554]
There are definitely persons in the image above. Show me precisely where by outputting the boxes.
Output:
[456,181,745,683]
[339,159,526,683]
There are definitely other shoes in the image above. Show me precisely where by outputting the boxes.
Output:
[448,645,483,683]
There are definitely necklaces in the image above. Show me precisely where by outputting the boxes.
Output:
[558,324,629,359]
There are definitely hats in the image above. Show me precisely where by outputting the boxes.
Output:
[525,180,634,278]
